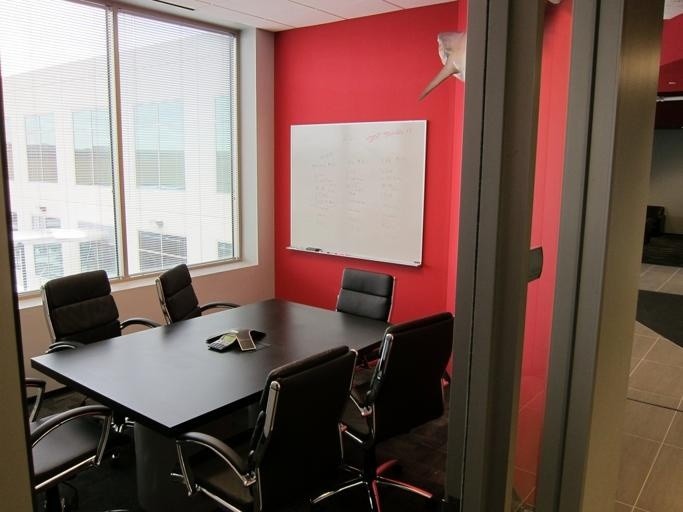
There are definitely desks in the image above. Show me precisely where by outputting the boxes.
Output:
[36,297,403,502]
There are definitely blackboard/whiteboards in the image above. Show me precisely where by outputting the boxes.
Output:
[285,120,428,268]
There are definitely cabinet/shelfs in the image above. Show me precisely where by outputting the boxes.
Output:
[654,0,683,129]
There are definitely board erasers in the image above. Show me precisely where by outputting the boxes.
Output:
[306,247,322,251]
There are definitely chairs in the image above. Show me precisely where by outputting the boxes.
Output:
[25,377,124,510]
[154,263,243,325]
[315,304,454,508]
[36,268,162,447]
[167,342,359,512]
[331,267,397,321]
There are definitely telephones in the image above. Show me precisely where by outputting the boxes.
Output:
[206,327,265,352]
